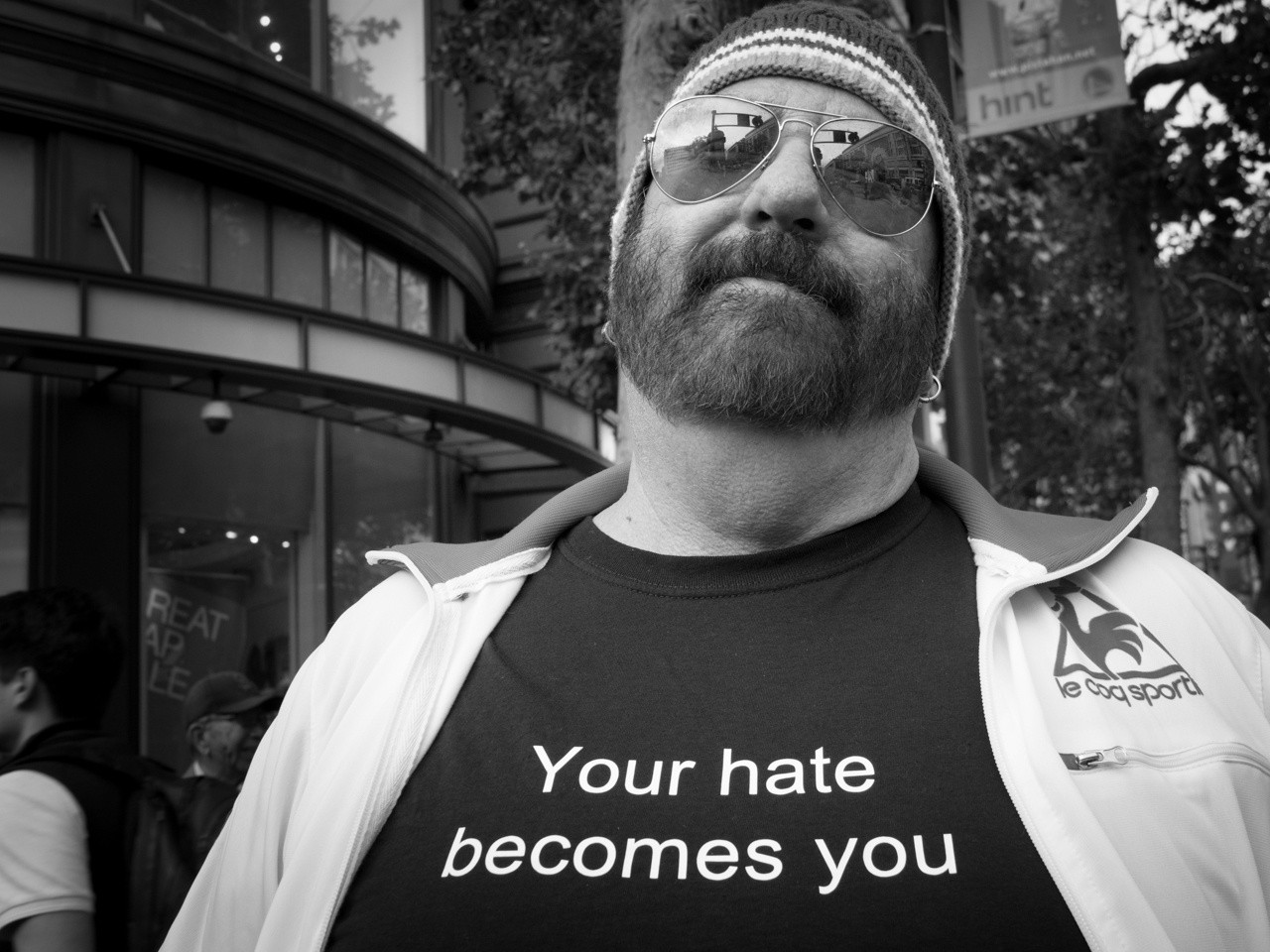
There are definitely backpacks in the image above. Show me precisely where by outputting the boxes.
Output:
[14,755,241,952]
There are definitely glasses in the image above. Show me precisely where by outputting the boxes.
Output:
[642,95,947,238]
[196,713,273,730]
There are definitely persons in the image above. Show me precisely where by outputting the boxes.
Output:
[0,590,124,952]
[146,671,281,952]
[159,0,1269,952]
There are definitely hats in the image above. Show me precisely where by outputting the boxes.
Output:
[610,0,973,382]
[184,671,284,730]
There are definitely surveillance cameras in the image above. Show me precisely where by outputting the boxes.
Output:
[201,400,233,433]
